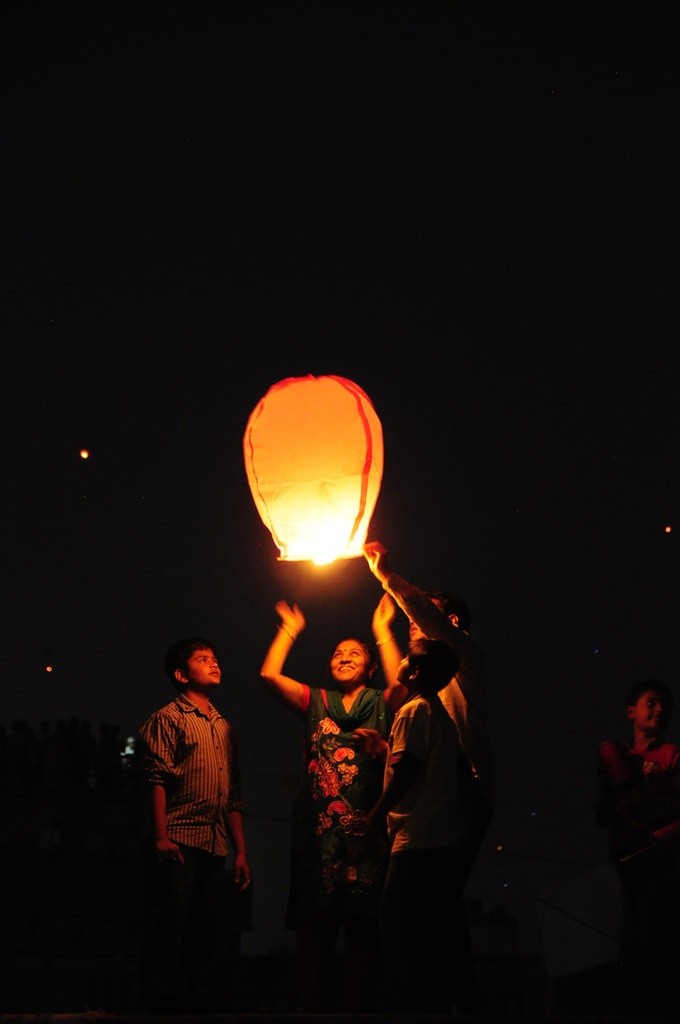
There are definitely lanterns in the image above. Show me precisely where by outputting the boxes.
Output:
[243,373,383,561]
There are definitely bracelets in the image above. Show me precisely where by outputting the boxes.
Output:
[376,635,392,646]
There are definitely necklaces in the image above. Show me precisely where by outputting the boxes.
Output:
[403,691,420,705]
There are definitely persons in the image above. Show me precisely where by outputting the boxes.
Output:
[260,541,523,1012]
[593,675,680,1007]
[133,638,251,1014]
